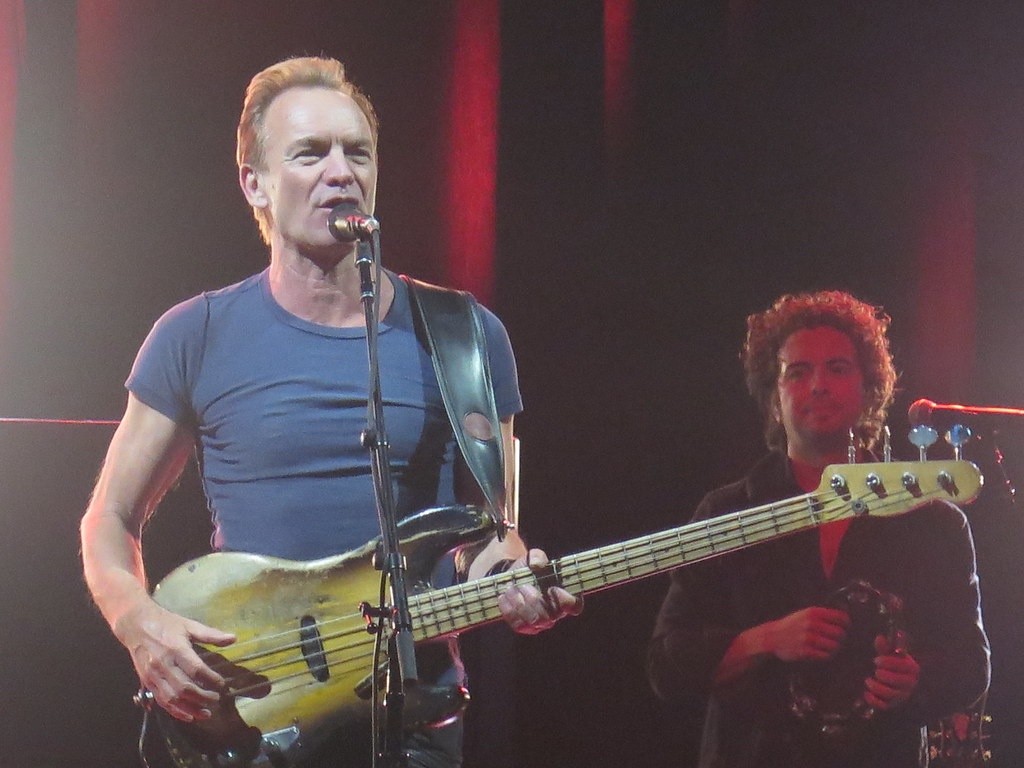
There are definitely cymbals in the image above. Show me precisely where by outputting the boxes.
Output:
[778,574,909,736]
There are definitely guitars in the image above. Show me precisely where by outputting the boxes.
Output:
[137,421,984,768]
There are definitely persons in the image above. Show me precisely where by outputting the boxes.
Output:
[79,57,584,768]
[645,291,991,768]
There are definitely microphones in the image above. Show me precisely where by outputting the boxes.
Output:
[327,203,380,242]
[908,399,1024,433]
